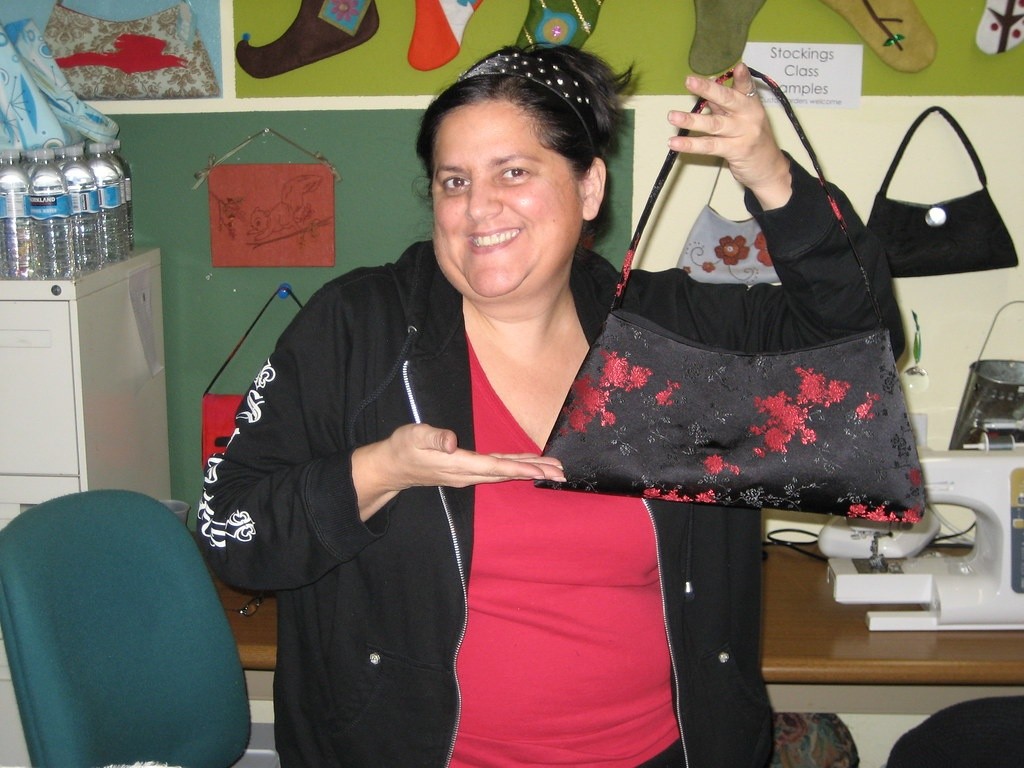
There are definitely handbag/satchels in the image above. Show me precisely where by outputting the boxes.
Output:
[530,68,928,523]
[678,147,785,284]
[43,1,221,100]
[866,104,1019,279]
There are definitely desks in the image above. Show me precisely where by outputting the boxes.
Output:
[204,543,1024,717]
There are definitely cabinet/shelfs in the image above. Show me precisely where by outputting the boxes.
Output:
[0,247,173,768]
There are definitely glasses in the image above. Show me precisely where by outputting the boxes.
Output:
[223,591,266,617]
[765,527,826,563]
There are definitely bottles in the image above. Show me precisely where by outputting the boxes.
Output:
[0,141,135,281]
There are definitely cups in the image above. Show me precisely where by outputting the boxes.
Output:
[159,500,190,525]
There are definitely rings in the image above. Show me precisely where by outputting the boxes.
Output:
[746,88,757,96]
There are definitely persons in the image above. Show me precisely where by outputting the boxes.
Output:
[195,40,907,768]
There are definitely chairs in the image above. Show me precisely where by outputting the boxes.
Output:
[1,488,252,768]
